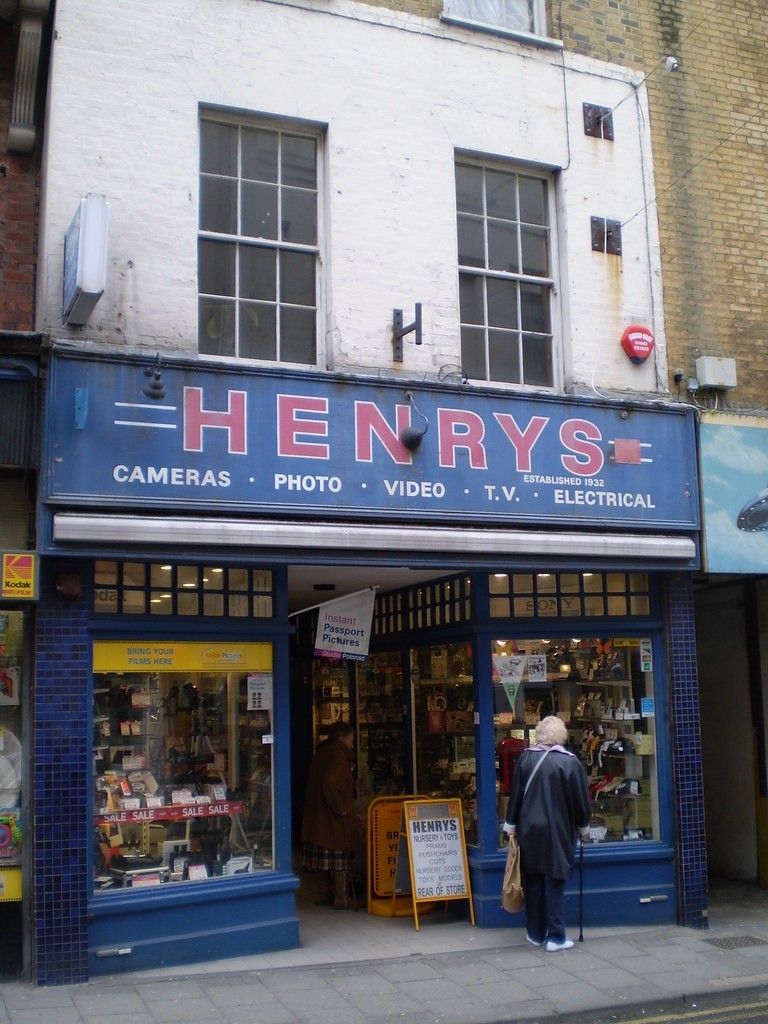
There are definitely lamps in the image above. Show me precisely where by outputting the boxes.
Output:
[143,353,166,398]
[402,392,430,450]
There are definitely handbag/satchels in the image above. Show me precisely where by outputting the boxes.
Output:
[501,834,526,914]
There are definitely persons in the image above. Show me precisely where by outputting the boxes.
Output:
[298,720,366,910]
[502,716,592,952]
[251,692,262,708]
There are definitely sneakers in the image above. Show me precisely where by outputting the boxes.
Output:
[546,941,574,952]
[527,934,546,946]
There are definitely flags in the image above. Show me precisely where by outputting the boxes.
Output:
[313,589,376,663]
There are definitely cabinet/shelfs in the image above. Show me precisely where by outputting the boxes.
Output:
[92,674,272,891]
[311,642,644,847]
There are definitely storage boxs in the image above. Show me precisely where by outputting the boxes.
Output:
[498,795,510,819]
[492,685,526,723]
[426,685,449,711]
[427,711,446,731]
[625,734,653,755]
[158,840,190,867]
[592,777,652,839]
[430,646,457,679]
[447,710,475,730]
[107,862,170,888]
